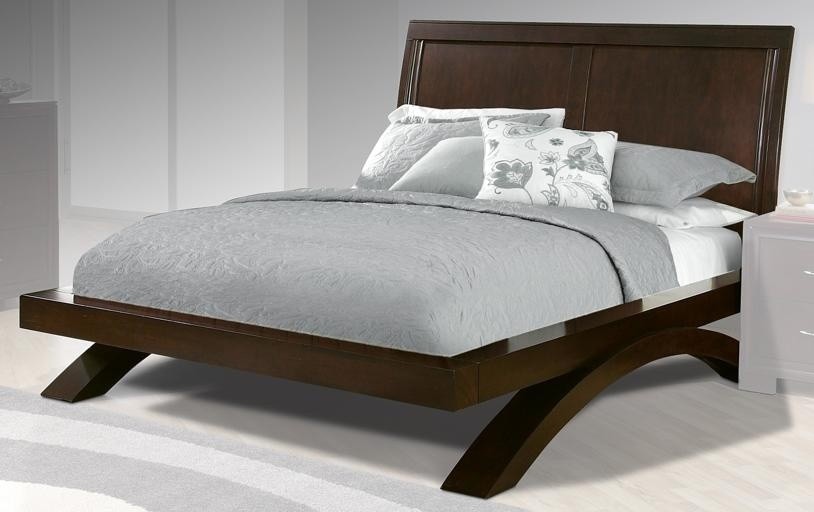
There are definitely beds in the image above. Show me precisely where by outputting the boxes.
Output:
[17,19,796,500]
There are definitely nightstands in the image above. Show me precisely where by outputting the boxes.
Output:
[737,204,814,398]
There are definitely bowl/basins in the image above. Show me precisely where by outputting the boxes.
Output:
[783,188,812,207]
